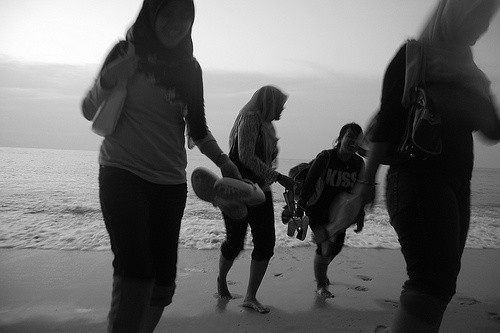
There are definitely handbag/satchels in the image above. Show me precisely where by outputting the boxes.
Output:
[91,40,136,136]
[354,40,427,165]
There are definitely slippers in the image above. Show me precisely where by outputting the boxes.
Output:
[214,178,266,205]
[287,218,299,236]
[297,215,309,240]
[191,166,247,219]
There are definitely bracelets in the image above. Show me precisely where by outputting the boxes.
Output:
[356,180,374,184]
[216,153,227,166]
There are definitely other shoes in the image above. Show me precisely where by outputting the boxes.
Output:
[326,195,363,236]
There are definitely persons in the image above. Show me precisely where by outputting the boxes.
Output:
[217,88,297,313]
[355,0,500,333]
[84,0,241,333]
[295,123,365,298]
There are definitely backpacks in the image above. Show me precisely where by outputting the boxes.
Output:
[282,149,331,212]
